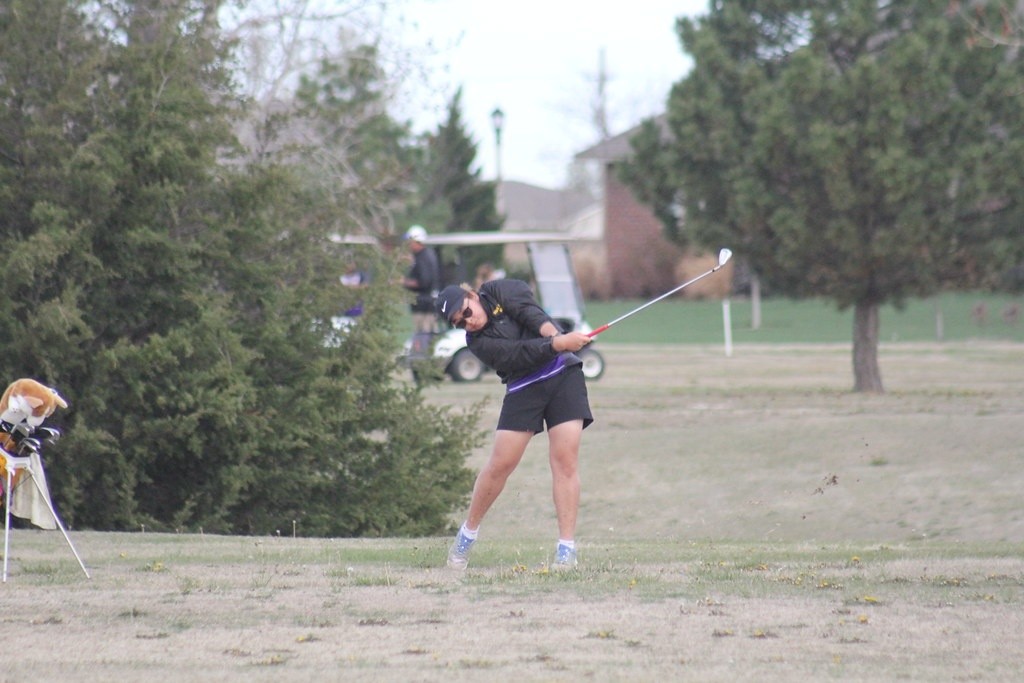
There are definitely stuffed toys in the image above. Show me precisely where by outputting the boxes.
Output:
[0,378,68,428]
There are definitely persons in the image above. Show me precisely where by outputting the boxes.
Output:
[393,225,442,390]
[436,278,594,573]
[474,265,505,290]
[336,251,366,318]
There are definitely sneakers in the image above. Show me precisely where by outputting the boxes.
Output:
[551,542,578,569]
[447,525,477,571]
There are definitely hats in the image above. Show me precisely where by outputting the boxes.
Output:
[437,285,469,322]
[402,225,427,245]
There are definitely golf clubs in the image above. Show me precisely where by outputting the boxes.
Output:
[586,247,733,338]
[1,422,60,457]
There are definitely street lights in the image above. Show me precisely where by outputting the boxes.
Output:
[490,108,504,176]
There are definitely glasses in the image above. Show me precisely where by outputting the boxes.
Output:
[451,298,473,329]
